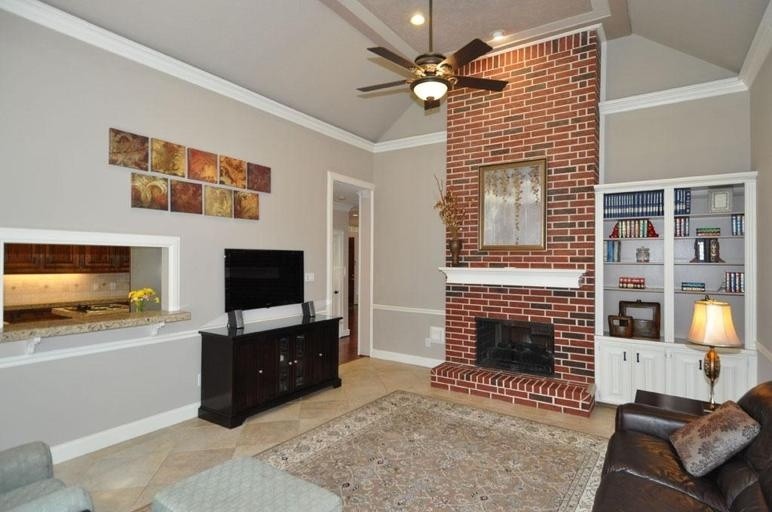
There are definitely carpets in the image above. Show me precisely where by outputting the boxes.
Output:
[253,389,610,512]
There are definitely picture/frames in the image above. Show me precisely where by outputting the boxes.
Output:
[477,157,548,252]
[706,187,734,212]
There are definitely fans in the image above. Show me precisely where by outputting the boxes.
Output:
[357,3,505,93]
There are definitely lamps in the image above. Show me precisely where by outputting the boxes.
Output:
[406,76,455,103]
[348,206,361,237]
[688,299,740,403]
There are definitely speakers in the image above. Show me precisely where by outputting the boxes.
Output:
[301,301,315,316]
[227,310,244,329]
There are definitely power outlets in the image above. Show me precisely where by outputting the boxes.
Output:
[423,336,433,349]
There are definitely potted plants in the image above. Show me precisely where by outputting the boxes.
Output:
[433,173,470,267]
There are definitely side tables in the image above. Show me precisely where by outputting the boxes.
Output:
[635,390,722,420]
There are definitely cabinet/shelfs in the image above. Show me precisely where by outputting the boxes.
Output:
[591,169,759,335]
[3,244,84,274]
[88,244,131,274]
[594,338,664,406]
[198,314,344,428]
[665,344,753,411]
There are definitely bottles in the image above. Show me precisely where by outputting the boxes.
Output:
[635,247,649,262]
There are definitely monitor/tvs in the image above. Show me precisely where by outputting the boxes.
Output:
[224,248,304,312]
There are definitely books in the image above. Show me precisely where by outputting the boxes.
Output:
[603,240,619,262]
[731,214,743,237]
[617,219,647,238]
[683,287,705,292]
[726,270,743,292]
[618,276,644,283]
[696,232,720,236]
[695,227,720,233]
[682,281,703,286]
[674,189,690,214]
[603,191,662,217]
[619,284,644,289]
[674,217,687,237]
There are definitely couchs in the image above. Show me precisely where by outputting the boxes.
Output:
[148,455,343,511]
[1,435,95,512]
[591,380,770,510]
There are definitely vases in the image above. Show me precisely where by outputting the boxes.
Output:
[129,299,143,314]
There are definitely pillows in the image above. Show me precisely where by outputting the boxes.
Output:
[669,396,760,476]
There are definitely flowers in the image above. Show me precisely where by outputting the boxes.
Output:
[127,287,161,314]
